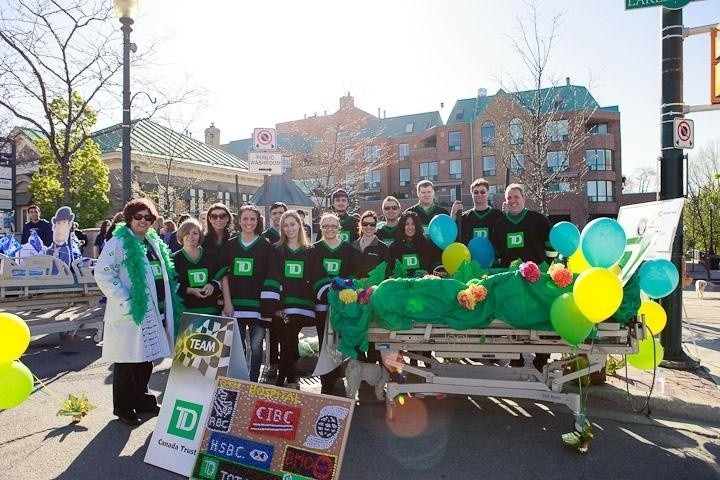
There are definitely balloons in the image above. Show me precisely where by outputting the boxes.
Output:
[0,312,31,366]
[547,217,680,369]
[0,363,34,412]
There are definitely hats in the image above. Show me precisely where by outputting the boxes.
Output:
[52,207,74,223]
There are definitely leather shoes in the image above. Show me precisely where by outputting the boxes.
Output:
[135,402,162,414]
[112,405,143,426]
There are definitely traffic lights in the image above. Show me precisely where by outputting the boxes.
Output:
[708,27,720,104]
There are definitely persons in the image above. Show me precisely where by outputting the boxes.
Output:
[20,205,50,246]
[156,178,552,387]
[91,198,176,425]
[48,207,74,270]
[72,212,124,261]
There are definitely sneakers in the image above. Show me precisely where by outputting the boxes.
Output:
[267,363,347,392]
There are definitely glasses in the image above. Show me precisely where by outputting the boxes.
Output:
[207,210,229,220]
[360,220,377,227]
[321,224,339,230]
[383,205,400,211]
[128,211,156,223]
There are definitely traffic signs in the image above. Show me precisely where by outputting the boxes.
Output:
[622,0,698,12]
[246,151,283,177]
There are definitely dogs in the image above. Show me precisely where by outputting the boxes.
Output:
[344,357,391,403]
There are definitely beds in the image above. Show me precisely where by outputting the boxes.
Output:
[0,256,107,343]
[326,237,651,453]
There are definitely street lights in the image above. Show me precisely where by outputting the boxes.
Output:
[109,0,141,205]
[683,224,689,259]
[708,201,716,255]
